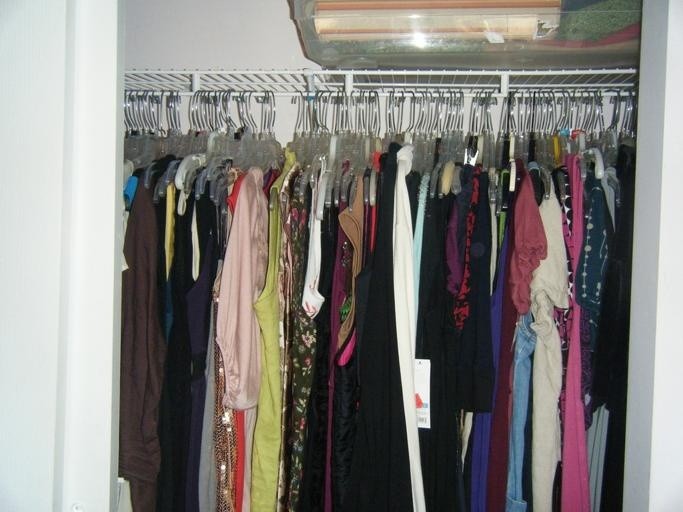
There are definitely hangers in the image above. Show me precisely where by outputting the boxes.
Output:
[123,89,634,214]
